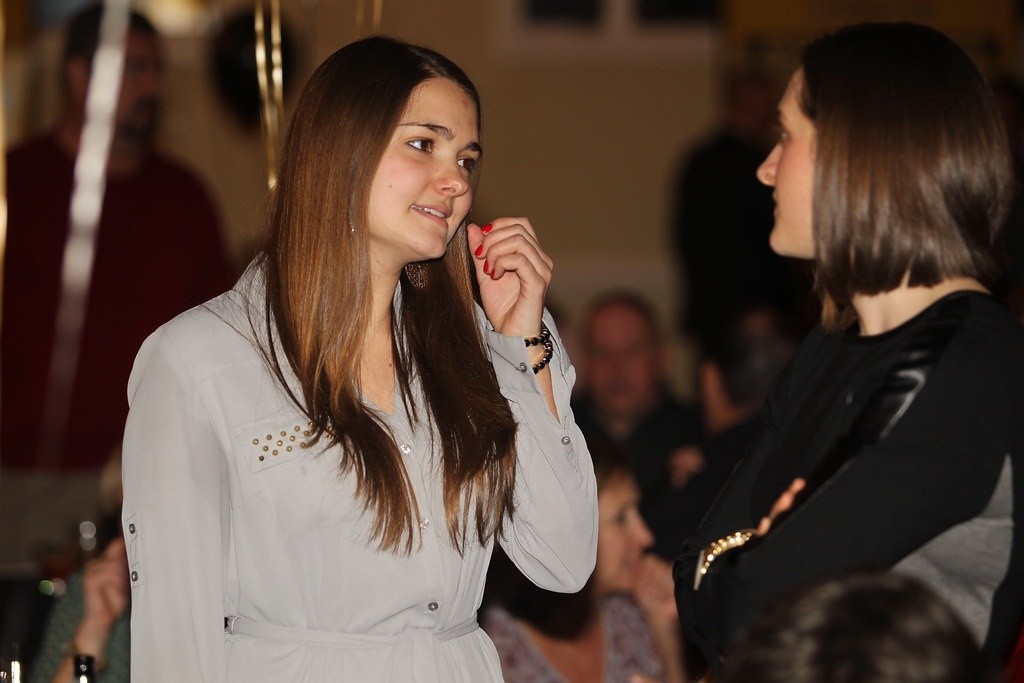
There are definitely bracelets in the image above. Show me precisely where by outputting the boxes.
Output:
[523,322,553,372]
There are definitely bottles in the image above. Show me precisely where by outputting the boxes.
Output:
[0,640,21,683]
[69,654,96,683]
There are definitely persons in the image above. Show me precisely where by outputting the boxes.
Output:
[671,20,1024,683]
[26,506,132,683]
[469,288,712,683]
[0,0,235,683]
[121,35,600,683]
